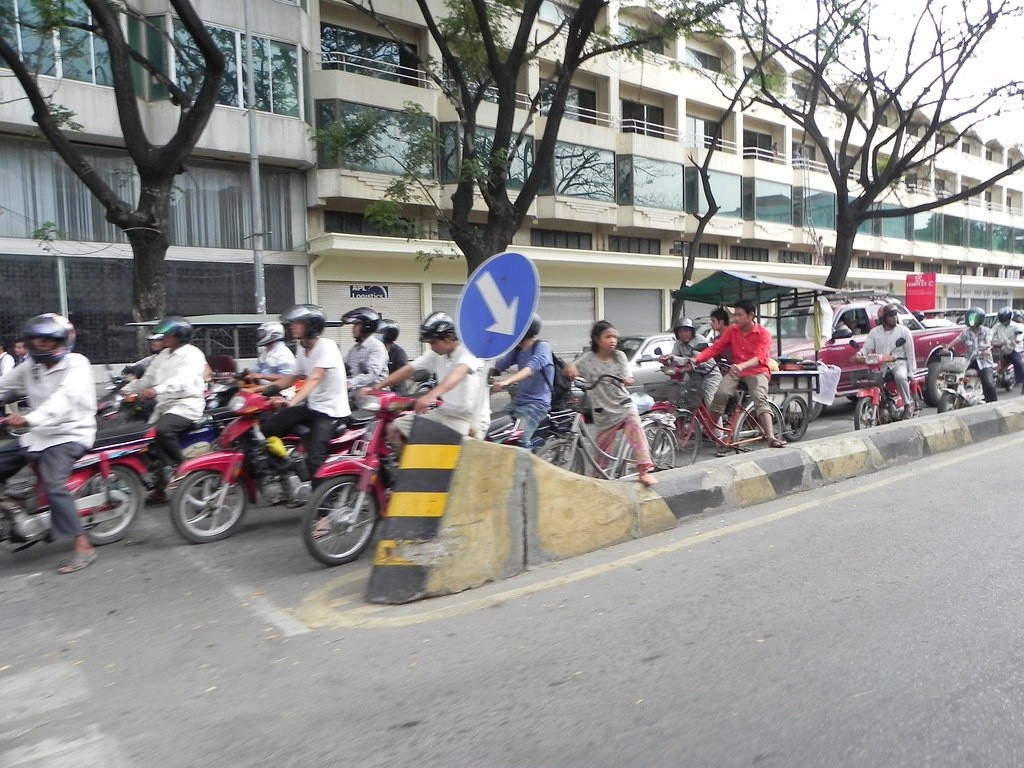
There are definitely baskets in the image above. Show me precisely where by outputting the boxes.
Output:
[941,356,967,372]
[643,379,705,408]
[850,370,883,388]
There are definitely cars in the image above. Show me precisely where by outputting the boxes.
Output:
[562,335,685,416]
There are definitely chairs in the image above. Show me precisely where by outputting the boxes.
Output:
[846,320,862,335]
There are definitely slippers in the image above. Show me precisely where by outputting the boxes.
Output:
[771,439,788,447]
[311,524,333,539]
[716,452,727,457]
[58,551,99,572]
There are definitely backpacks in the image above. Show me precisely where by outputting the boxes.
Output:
[514,340,573,411]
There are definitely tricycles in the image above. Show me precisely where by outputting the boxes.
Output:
[641,271,841,459]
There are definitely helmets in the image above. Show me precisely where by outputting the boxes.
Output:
[256,321,285,346]
[673,316,695,340]
[912,310,924,321]
[151,315,195,345]
[145,334,168,354]
[878,304,899,327]
[279,304,326,339]
[22,313,76,364]
[998,306,1024,325]
[523,313,542,339]
[420,311,459,342]
[341,307,381,333]
[965,307,985,327]
[376,319,399,343]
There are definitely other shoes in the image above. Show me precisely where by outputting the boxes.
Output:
[639,473,657,486]
[145,490,171,504]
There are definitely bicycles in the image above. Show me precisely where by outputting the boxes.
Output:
[535,357,676,482]
[653,356,795,472]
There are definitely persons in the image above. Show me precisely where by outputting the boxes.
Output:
[487,314,555,448]
[655,300,787,448]
[355,311,491,463]
[341,306,410,428]
[989,306,1024,395]
[853,303,918,420]
[241,304,351,540]
[940,306,998,403]
[119,315,206,504]
[562,320,658,485]
[0,339,30,415]
[244,321,296,402]
[0,313,100,574]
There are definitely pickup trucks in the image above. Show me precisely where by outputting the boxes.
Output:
[757,292,970,423]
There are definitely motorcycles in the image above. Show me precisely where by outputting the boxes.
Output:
[984,330,1023,393]
[0,388,159,555]
[95,363,582,569]
[848,336,924,432]
[937,345,998,415]
